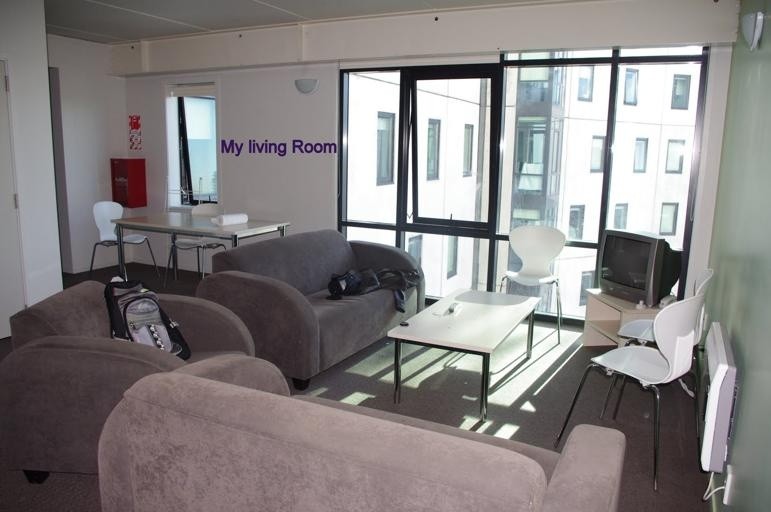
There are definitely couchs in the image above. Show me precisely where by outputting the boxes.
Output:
[195,230,426,391]
[98,352,627,512]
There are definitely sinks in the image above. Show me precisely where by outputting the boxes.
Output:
[192,203,221,215]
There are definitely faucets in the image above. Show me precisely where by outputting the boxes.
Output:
[208,194,212,202]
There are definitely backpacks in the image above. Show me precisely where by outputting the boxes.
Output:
[103,276,191,368]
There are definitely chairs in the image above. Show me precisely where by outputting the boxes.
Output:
[0,280,255,485]
[554,291,705,492]
[598,269,716,443]
[500,225,566,345]
[90,201,162,280]
[163,204,226,289]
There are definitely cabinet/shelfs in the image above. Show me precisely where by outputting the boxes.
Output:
[582,288,661,349]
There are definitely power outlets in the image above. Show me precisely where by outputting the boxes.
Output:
[722,464,731,505]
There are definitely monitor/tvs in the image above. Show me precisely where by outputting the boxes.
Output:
[598,228,682,307]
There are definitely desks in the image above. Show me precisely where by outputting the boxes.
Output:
[110,210,291,281]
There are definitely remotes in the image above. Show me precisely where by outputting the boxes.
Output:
[448,302,459,312]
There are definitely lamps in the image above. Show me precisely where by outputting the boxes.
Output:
[296,79,318,93]
[741,12,764,52]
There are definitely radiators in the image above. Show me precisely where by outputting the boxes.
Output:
[694,322,737,473]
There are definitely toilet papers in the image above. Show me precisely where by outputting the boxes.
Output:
[211,213,248,225]
